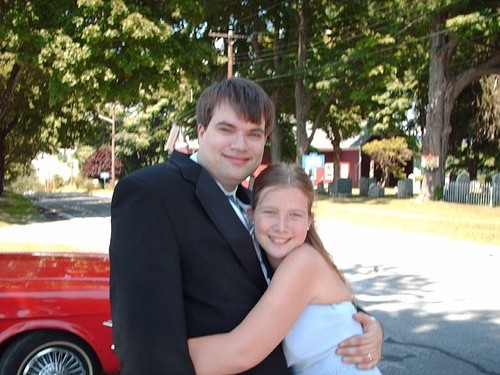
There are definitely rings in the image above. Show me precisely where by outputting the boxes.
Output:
[368,352,372,363]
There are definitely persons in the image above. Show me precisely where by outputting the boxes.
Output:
[109,78,384,375]
[187,162,382,375]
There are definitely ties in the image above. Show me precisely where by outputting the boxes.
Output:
[228,195,271,286]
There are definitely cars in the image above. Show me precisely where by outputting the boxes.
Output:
[1,252,122,375]
